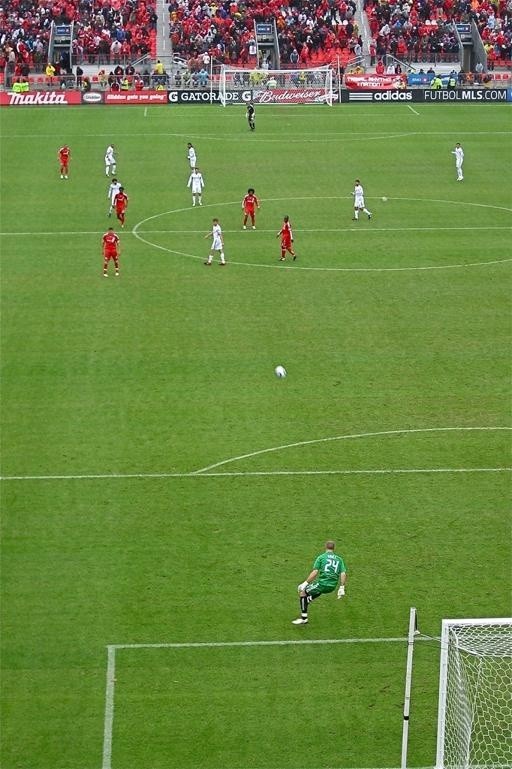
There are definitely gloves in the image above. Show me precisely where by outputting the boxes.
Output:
[297,580,309,594]
[337,586,345,600]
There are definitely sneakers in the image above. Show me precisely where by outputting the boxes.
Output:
[192,201,203,207]
[352,218,358,220]
[251,225,256,229]
[217,262,226,265]
[457,176,463,180]
[107,172,115,176]
[60,174,67,179]
[367,214,371,220]
[103,272,119,277]
[292,618,309,625]
[292,254,297,261]
[243,226,247,230]
[204,261,212,265]
[106,213,112,218]
[278,257,285,261]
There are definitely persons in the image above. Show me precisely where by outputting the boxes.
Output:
[275,215,296,262]
[241,189,260,230]
[0,0,512,91]
[187,142,197,171]
[57,144,71,179]
[205,219,227,266]
[100,144,128,277]
[186,167,205,207]
[245,103,256,129]
[291,541,347,626]
[452,143,464,181]
[350,179,373,220]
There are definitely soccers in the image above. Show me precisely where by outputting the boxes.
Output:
[274,365,286,377]
